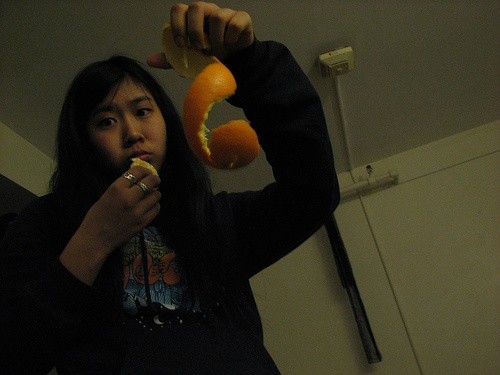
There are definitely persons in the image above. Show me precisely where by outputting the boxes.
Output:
[2,1,341,374]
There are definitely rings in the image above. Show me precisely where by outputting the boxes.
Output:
[122,172,137,185]
[137,181,151,195]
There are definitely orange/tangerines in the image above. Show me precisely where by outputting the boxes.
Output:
[130,157,159,177]
